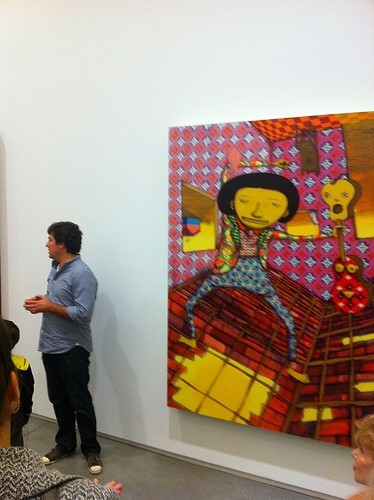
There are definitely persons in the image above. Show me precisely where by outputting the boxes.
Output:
[0,317,124,500]
[21,221,104,474]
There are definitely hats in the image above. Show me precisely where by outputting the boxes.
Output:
[0,318,20,350]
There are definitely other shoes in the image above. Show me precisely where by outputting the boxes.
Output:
[87,455,103,474]
[41,448,75,464]
